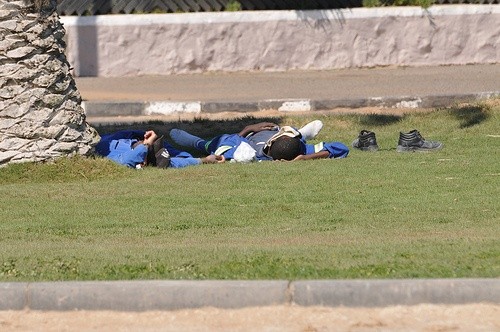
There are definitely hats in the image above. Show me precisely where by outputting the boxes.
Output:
[146,134,170,169]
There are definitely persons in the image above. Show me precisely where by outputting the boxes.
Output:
[93,129,226,171]
[170,116,350,164]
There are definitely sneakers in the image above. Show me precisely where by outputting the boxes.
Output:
[397,129,442,152]
[353,129,379,152]
